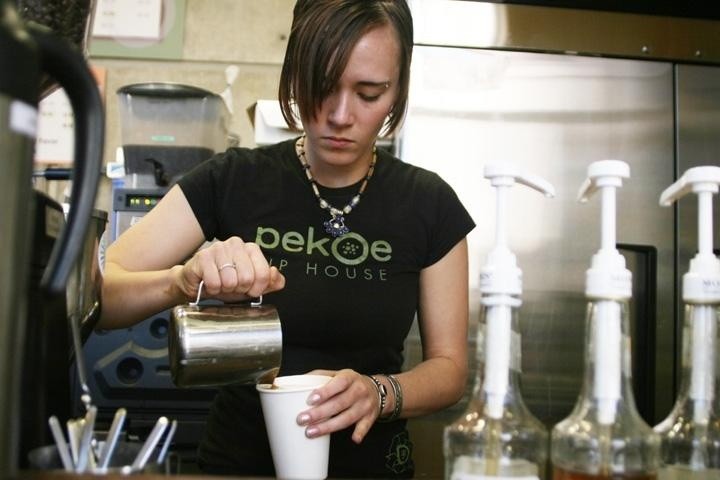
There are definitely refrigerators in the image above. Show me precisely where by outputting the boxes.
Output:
[404,43,720,480]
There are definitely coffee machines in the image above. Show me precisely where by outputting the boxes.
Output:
[76,78,236,461]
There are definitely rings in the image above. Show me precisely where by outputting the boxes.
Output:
[216,263,236,272]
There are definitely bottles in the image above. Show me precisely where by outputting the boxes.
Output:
[443,163,555,477]
[546,158,662,479]
[650,164,718,480]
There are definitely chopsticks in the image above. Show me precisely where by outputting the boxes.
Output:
[47,413,178,473]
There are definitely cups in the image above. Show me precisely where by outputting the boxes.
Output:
[168,281,283,390]
[0,8,106,480]
[254,377,332,480]
[26,447,174,480]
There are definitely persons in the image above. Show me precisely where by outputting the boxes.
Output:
[94,0,476,480]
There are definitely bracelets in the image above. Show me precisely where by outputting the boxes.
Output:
[367,375,388,421]
[378,373,403,428]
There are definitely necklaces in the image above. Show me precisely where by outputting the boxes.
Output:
[297,131,378,238]
